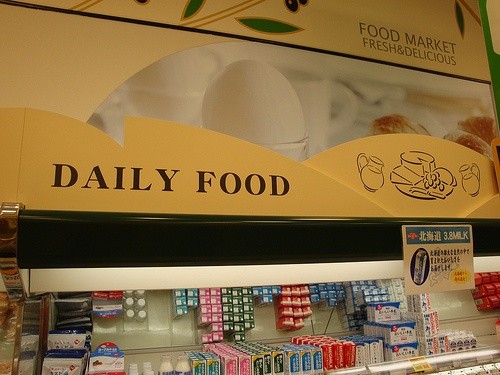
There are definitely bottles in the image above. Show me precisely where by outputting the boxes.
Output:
[158,354,174,375]
[174,355,191,375]
[127,360,155,375]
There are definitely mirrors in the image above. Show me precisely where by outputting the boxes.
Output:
[48,270,500,350]
